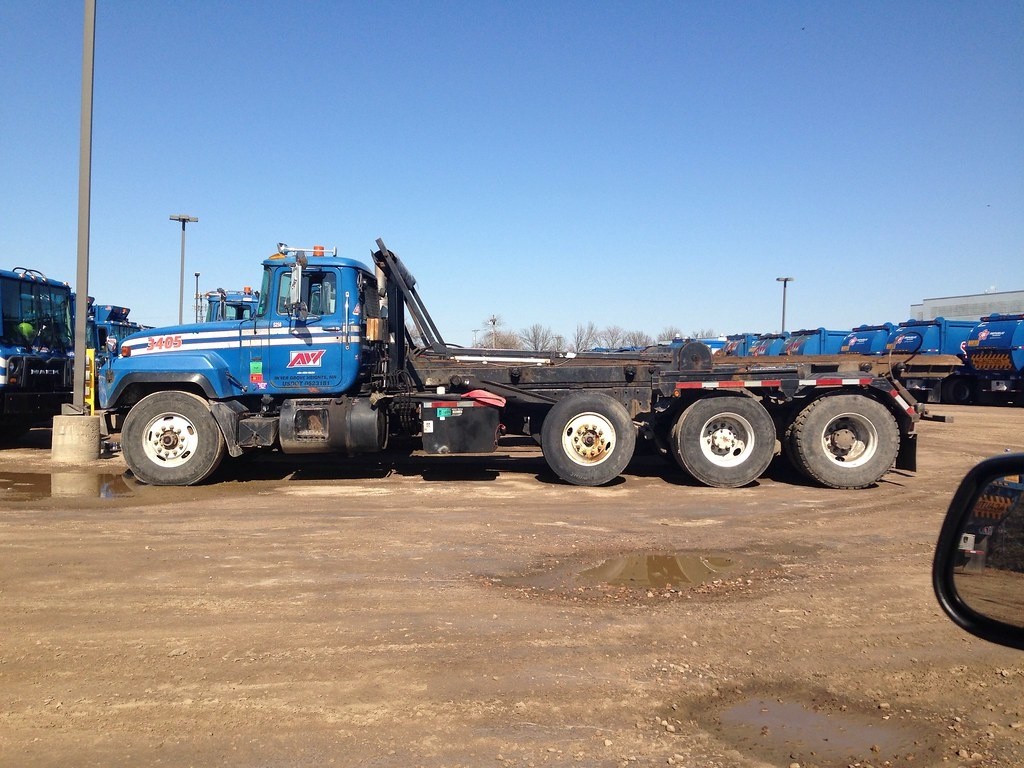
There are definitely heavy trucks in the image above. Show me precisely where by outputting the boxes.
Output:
[98,248,965,490]
[0,267,155,450]
[206,287,291,323]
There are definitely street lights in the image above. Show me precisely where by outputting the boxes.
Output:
[472,329,480,348]
[776,277,793,333]
[195,272,200,323]
[169,214,198,325]
[490,315,497,349]
